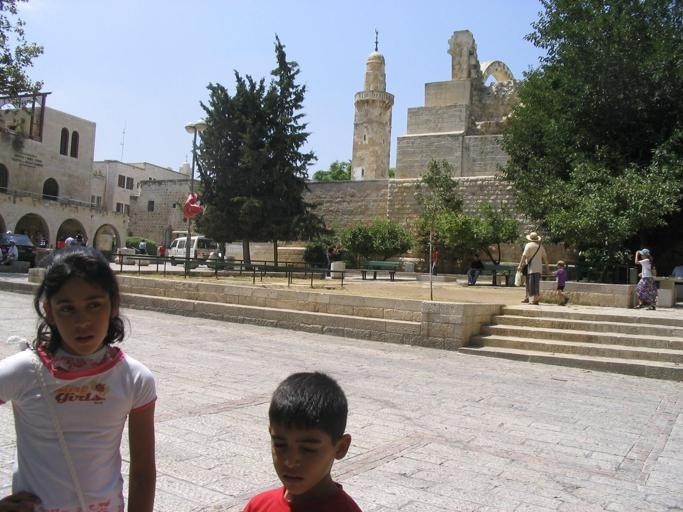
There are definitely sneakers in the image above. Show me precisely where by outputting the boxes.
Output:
[558,297,569,306]
[521,298,539,305]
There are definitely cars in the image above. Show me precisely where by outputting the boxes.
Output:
[0,233,36,268]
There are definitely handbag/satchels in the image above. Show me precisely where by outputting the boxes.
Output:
[521,263,528,276]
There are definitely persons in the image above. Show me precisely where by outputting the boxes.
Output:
[139,239,146,252]
[327,244,337,277]
[547,260,569,306]
[517,232,550,305]
[243,372,363,512]
[468,254,484,286]
[2,244,158,511]
[432,247,440,275]
[156,240,169,256]
[0,231,86,265]
[633,248,657,310]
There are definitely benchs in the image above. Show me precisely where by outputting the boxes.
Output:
[472,264,514,285]
[360,261,400,281]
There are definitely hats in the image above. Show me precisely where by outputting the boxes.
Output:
[9,239,16,244]
[526,232,541,241]
[557,260,564,267]
[639,248,650,256]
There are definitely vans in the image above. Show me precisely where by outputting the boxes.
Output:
[168,236,209,268]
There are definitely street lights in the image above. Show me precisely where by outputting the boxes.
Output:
[185,121,207,277]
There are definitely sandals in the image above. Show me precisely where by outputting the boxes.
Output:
[633,303,644,309]
[646,304,656,310]
